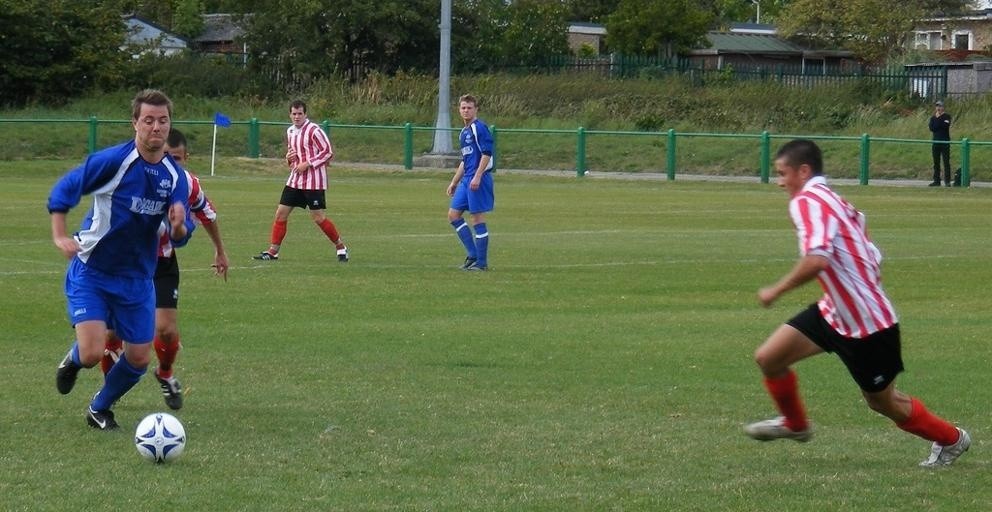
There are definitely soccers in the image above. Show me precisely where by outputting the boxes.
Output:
[135,412,186,462]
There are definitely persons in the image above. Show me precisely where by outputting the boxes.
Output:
[741,138,973,468]
[250,99,351,262]
[103,131,228,411]
[47,88,195,431]
[447,94,494,271]
[929,102,952,187]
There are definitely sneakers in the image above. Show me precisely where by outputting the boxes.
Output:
[929,182,940,185]
[154,368,183,409]
[337,246,349,263]
[746,417,810,442]
[460,256,487,271]
[87,405,119,431]
[253,251,279,260]
[919,428,970,467]
[56,350,80,394]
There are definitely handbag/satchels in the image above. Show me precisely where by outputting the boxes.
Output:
[953,168,961,186]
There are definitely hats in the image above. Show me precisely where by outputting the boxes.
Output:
[936,99,944,106]
